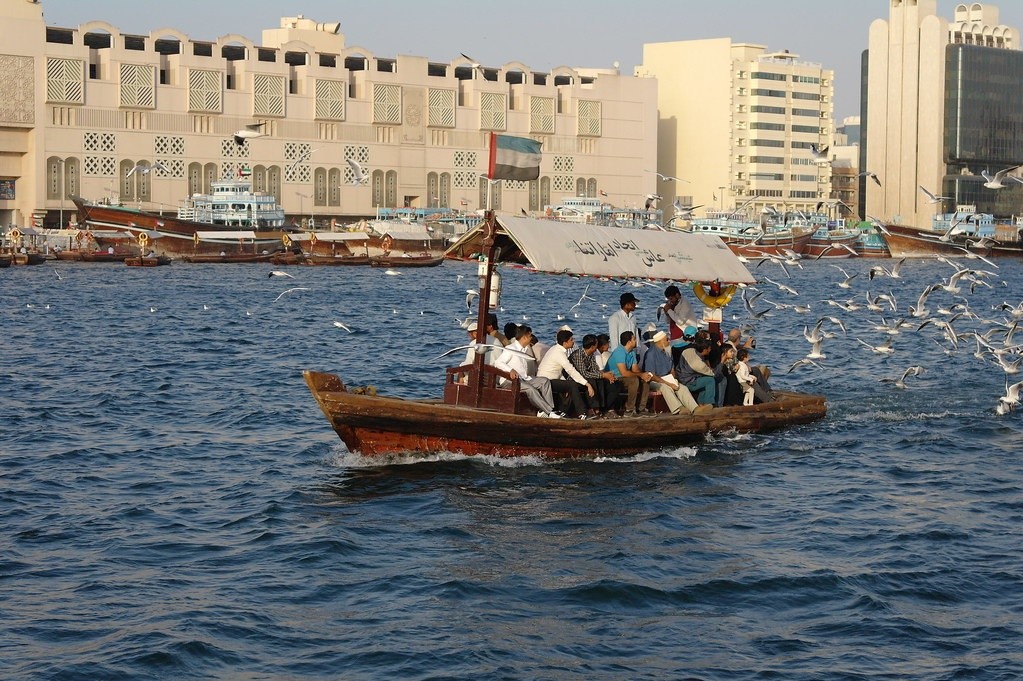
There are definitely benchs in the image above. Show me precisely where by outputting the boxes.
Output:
[444,363,700,415]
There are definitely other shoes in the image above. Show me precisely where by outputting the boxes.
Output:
[624,410,643,419]
[578,413,592,420]
[607,411,621,418]
[679,409,690,415]
[597,412,608,420]
[639,410,657,417]
[588,409,598,419]
[693,404,713,413]
[558,411,570,417]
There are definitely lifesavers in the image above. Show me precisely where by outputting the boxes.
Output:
[283,234,288,242]
[194,233,199,245]
[310,238,317,245]
[139,233,148,240]
[139,240,148,246]
[310,232,314,240]
[300,239,309,248]
[12,230,20,238]
[693,281,738,308]
[382,242,389,250]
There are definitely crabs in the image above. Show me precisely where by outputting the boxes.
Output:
[273,287,310,302]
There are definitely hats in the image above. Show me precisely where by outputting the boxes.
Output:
[653,331,666,342]
[620,292,640,302]
[468,323,478,331]
[684,326,697,338]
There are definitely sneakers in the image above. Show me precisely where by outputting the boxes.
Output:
[549,412,561,419]
[537,411,549,418]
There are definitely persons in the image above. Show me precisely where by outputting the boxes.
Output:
[458,322,504,386]
[51,245,62,253]
[643,331,713,415]
[609,293,641,348]
[485,313,511,347]
[562,334,623,418]
[676,337,740,408]
[537,330,594,420]
[335,251,342,257]
[495,325,562,419]
[664,286,697,339]
[402,251,409,257]
[20,245,26,253]
[504,322,742,367]
[723,328,775,406]
[603,331,657,418]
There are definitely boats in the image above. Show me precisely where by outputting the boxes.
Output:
[303,130,827,457]
[0,181,1023,267]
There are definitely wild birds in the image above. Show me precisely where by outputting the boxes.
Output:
[150,308,156,312]
[203,304,208,309]
[231,123,270,145]
[126,162,170,178]
[292,148,322,170]
[26,269,64,309]
[247,311,251,315]
[810,143,830,162]
[454,317,478,329]
[339,157,370,189]
[476,174,502,184]
[269,270,294,279]
[456,274,479,310]
[500,164,1023,414]
[418,311,434,315]
[378,268,402,276]
[429,343,537,362]
[461,52,490,83]
[333,320,351,334]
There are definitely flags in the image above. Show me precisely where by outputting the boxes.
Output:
[239,168,252,176]
[33,222,43,227]
[31,213,43,218]
[488,131,543,181]
